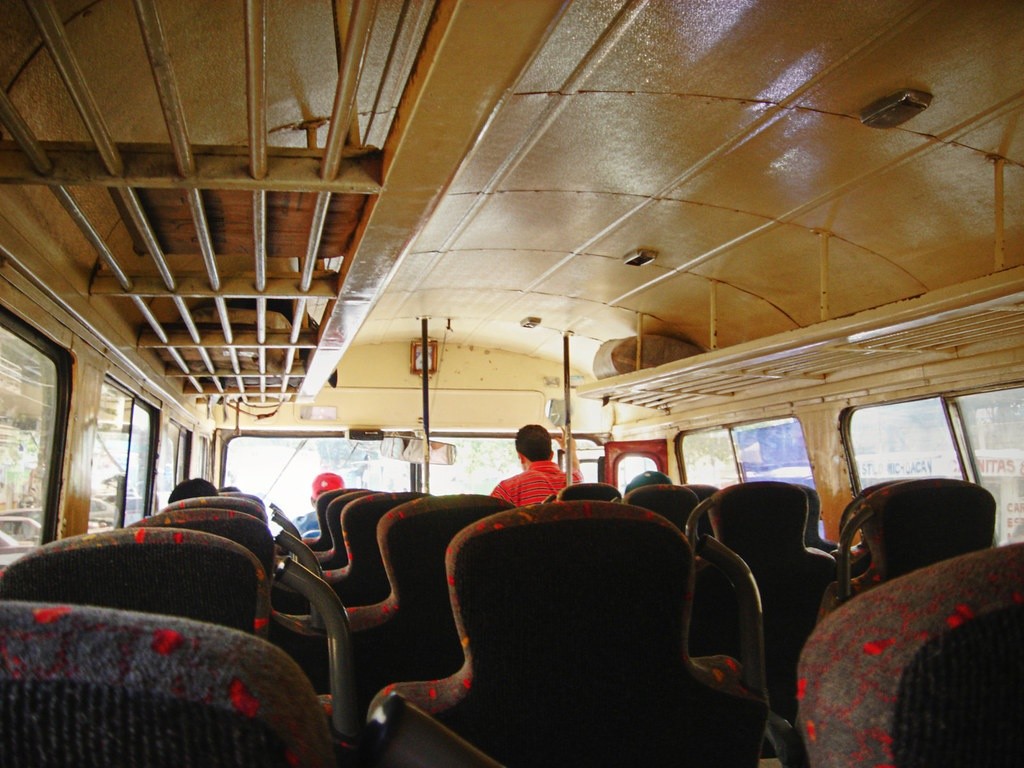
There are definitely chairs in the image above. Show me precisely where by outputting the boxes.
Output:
[0,476,1024,768]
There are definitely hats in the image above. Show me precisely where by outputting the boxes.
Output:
[311,473,345,499]
[626,470,673,495]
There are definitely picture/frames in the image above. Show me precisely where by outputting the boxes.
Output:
[411,339,439,374]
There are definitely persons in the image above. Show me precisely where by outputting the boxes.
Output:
[167,478,220,504]
[291,473,345,535]
[490,425,585,507]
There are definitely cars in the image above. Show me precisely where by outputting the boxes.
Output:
[0,494,171,568]
